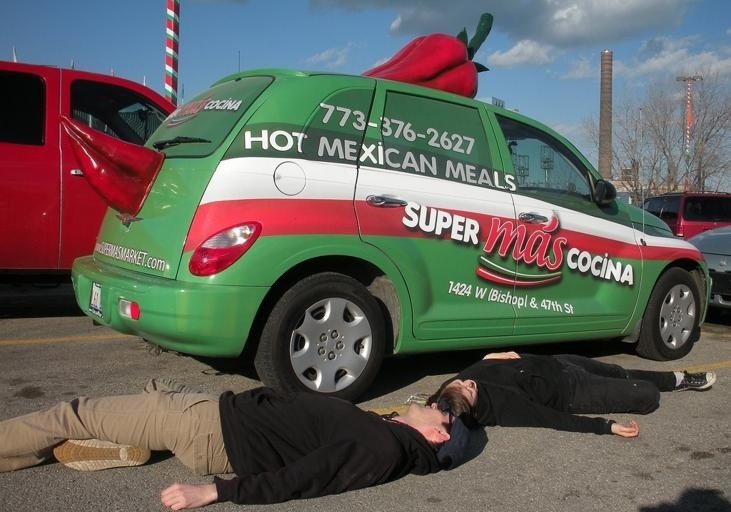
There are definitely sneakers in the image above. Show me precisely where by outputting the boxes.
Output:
[673,371,716,392]
[55,438,152,471]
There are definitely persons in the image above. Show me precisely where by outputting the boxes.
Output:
[0,377,471,510]
[425,351,717,438]
